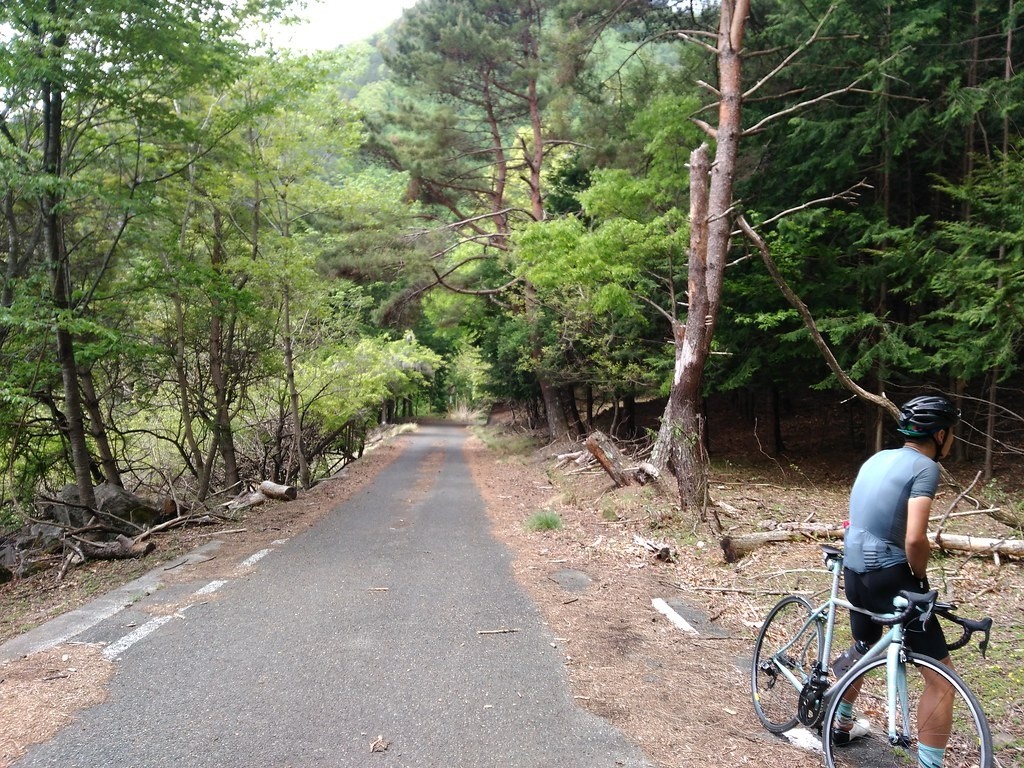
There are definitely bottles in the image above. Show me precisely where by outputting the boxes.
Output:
[833,640,869,679]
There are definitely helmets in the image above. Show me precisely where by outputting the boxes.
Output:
[899,396,963,445]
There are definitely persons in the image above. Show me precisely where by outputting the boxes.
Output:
[832,396,961,768]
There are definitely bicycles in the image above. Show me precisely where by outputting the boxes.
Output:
[751,545,995,768]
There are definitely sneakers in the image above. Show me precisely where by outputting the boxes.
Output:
[833,719,870,743]
